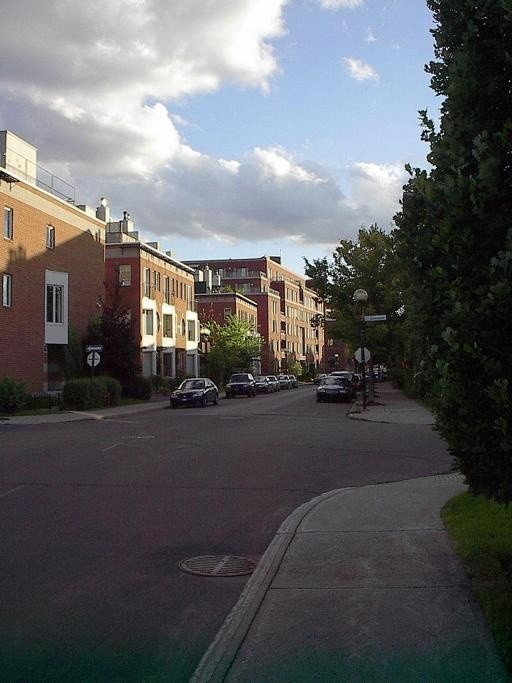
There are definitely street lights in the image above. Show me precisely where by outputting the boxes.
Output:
[334,353,339,371]
[200,327,211,378]
[351,289,369,410]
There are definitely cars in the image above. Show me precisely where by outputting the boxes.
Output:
[253,374,298,394]
[314,363,389,401]
[169,376,219,408]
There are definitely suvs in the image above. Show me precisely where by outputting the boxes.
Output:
[225,374,257,398]
